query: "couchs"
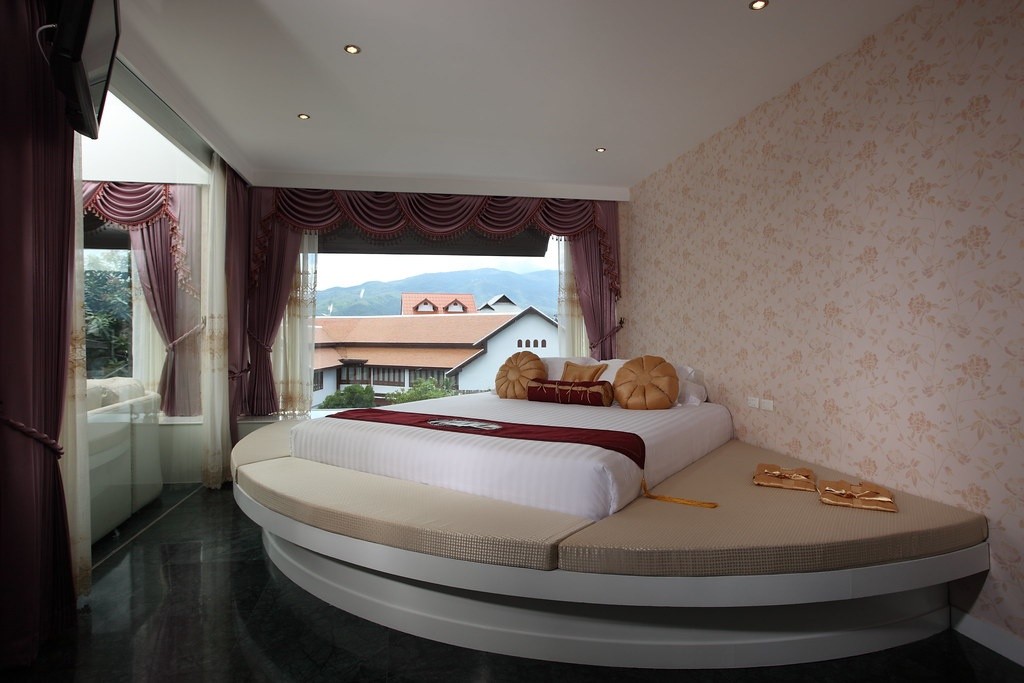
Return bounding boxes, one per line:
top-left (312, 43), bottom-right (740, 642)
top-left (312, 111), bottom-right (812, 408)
top-left (87, 376), bottom-right (161, 544)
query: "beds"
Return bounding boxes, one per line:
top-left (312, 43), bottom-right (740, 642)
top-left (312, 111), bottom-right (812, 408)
top-left (230, 354), bottom-right (991, 669)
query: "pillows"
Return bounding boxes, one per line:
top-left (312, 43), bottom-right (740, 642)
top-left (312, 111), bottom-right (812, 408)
top-left (613, 355), bottom-right (680, 409)
top-left (526, 378), bottom-right (614, 407)
top-left (495, 351), bottom-right (546, 399)
top-left (560, 361), bottom-right (608, 383)
